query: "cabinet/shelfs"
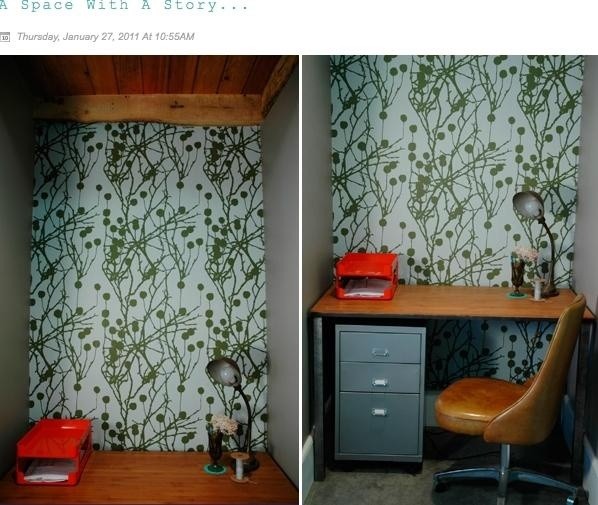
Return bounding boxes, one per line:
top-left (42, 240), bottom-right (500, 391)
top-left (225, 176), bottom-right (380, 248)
top-left (332, 325), bottom-right (426, 473)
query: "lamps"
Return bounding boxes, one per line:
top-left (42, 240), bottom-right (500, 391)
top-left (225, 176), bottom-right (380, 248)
top-left (511, 190), bottom-right (560, 299)
top-left (205, 358), bottom-right (261, 471)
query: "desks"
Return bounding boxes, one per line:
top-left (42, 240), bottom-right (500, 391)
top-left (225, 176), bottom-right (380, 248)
top-left (308, 275), bottom-right (595, 490)
top-left (1, 452), bottom-right (300, 505)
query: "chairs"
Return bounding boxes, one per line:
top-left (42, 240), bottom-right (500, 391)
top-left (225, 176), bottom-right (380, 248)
top-left (430, 288), bottom-right (593, 503)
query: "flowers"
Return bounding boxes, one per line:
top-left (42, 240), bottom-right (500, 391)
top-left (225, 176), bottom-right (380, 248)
top-left (512, 244), bottom-right (539, 266)
top-left (208, 414), bottom-right (238, 436)
